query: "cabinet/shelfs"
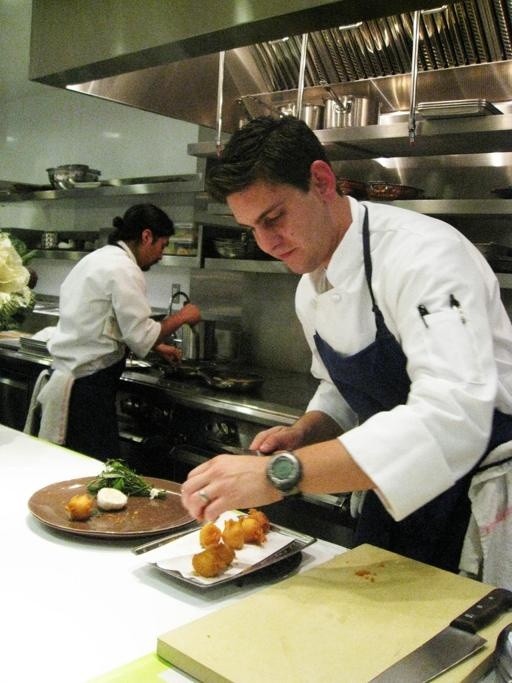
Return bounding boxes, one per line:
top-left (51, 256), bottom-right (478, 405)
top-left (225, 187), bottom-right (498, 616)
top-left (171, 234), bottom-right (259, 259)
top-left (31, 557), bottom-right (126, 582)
top-left (29, 0), bottom-right (511, 296)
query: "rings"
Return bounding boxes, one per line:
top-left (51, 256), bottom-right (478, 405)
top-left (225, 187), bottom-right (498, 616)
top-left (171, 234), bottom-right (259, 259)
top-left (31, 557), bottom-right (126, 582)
top-left (199, 491), bottom-right (211, 502)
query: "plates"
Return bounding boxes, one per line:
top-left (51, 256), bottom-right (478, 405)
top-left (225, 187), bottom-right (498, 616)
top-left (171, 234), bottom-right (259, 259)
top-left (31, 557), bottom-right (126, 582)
top-left (129, 512), bottom-right (318, 591)
top-left (73, 182), bottom-right (100, 190)
top-left (26, 475), bottom-right (207, 537)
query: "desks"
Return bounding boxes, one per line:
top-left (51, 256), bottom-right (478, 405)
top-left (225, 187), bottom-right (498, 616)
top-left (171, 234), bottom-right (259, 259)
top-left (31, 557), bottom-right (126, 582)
top-left (0, 421), bottom-right (512, 683)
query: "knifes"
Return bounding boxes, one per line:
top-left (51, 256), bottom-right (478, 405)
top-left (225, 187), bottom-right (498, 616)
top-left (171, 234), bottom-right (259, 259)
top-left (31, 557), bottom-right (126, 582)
top-left (367, 588), bottom-right (512, 683)
top-left (220, 446), bottom-right (273, 458)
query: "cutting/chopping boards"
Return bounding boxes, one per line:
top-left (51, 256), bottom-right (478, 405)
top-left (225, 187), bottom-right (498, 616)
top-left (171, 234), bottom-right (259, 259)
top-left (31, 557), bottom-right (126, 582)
top-left (157, 543), bottom-right (512, 683)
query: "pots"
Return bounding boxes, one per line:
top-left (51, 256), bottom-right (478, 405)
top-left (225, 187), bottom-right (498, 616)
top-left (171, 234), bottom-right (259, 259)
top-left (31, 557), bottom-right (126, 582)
top-left (369, 181), bottom-right (424, 201)
top-left (151, 357), bottom-right (265, 392)
top-left (243, 80), bottom-right (378, 130)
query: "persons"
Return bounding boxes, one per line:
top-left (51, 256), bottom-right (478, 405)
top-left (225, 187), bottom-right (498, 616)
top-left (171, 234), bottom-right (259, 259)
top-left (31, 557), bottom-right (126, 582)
top-left (178, 110), bottom-right (510, 596)
top-left (20, 202), bottom-right (204, 462)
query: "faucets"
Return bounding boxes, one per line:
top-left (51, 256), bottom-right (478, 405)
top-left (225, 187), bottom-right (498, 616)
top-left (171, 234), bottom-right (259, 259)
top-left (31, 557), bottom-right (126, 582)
top-left (169, 292), bottom-right (190, 316)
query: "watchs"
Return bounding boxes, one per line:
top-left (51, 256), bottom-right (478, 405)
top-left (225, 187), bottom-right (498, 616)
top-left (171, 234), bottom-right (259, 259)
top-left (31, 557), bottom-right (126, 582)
top-left (265, 450), bottom-right (304, 504)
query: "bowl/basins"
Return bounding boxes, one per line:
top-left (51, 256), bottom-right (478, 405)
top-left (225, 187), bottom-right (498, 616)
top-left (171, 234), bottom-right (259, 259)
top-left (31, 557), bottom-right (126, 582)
top-left (212, 236), bottom-right (272, 261)
top-left (44, 166), bottom-right (101, 190)
